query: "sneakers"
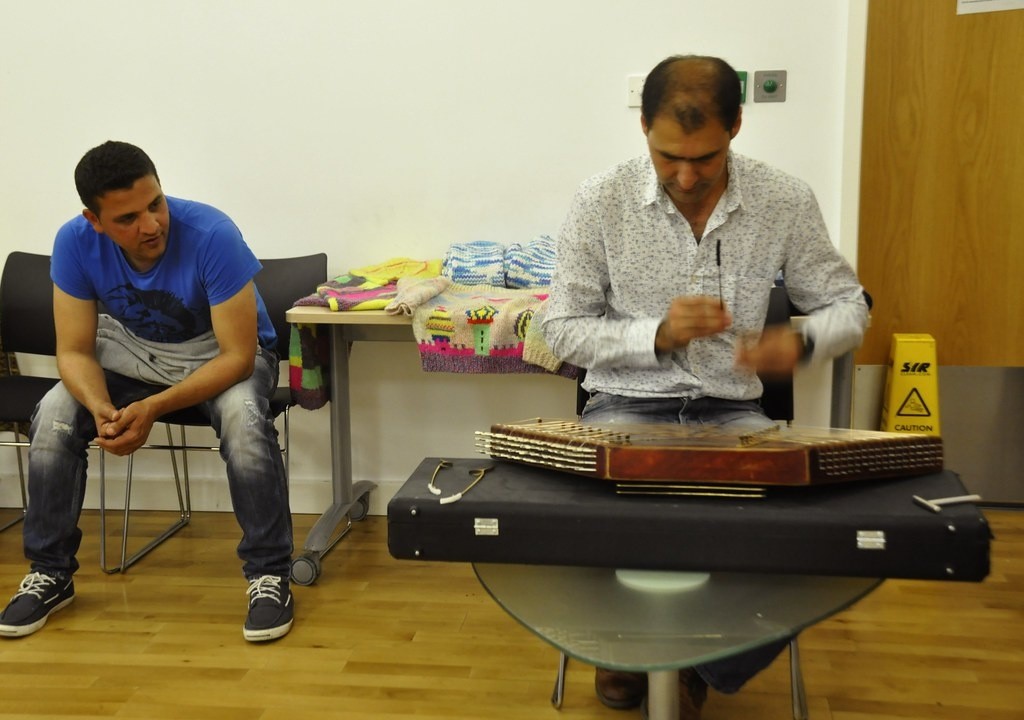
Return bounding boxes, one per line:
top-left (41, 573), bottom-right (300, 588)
top-left (0, 568), bottom-right (76, 637)
top-left (243, 576), bottom-right (295, 642)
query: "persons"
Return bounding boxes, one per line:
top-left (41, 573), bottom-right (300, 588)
top-left (1, 140), bottom-right (294, 641)
top-left (545, 54), bottom-right (871, 720)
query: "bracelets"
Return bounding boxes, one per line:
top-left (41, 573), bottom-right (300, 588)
top-left (800, 336), bottom-right (813, 363)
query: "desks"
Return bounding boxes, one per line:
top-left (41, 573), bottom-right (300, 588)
top-left (285, 306), bottom-right (856, 587)
top-left (473, 562), bottom-right (884, 720)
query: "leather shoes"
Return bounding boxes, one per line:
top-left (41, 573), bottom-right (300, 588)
top-left (595, 666), bottom-right (707, 720)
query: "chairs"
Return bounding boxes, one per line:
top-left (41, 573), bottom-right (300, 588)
top-left (553, 287), bottom-right (809, 720)
top-left (0, 252), bottom-right (185, 575)
top-left (119, 253), bottom-right (328, 574)
